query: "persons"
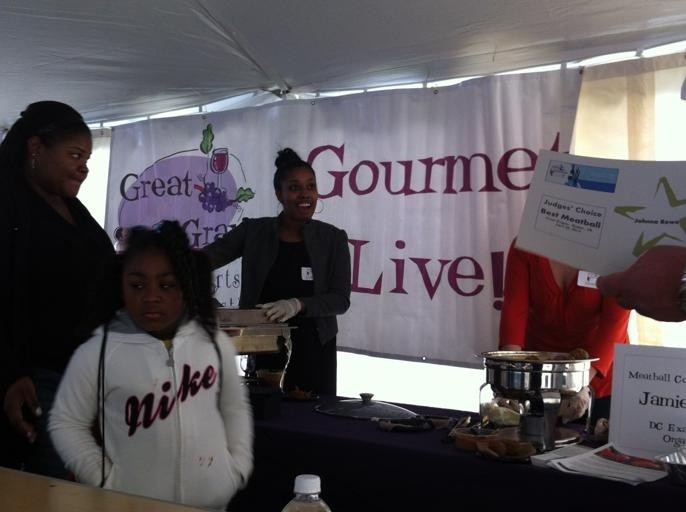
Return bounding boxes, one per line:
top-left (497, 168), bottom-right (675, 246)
top-left (597, 244), bottom-right (686, 323)
top-left (45, 218), bottom-right (255, 511)
top-left (0, 100), bottom-right (122, 483)
top-left (492, 232), bottom-right (632, 434)
top-left (201, 146), bottom-right (353, 396)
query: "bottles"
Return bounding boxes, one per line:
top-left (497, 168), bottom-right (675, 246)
top-left (282, 471), bottom-right (333, 512)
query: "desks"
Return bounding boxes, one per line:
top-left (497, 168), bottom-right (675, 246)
top-left (243, 376), bottom-right (686, 510)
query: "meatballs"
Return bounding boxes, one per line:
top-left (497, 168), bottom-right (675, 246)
top-left (569, 348), bottom-right (591, 360)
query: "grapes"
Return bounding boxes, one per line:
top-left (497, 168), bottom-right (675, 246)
top-left (199, 182), bottom-right (232, 213)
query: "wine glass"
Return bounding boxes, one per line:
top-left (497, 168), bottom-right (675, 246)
top-left (210, 147), bottom-right (229, 193)
